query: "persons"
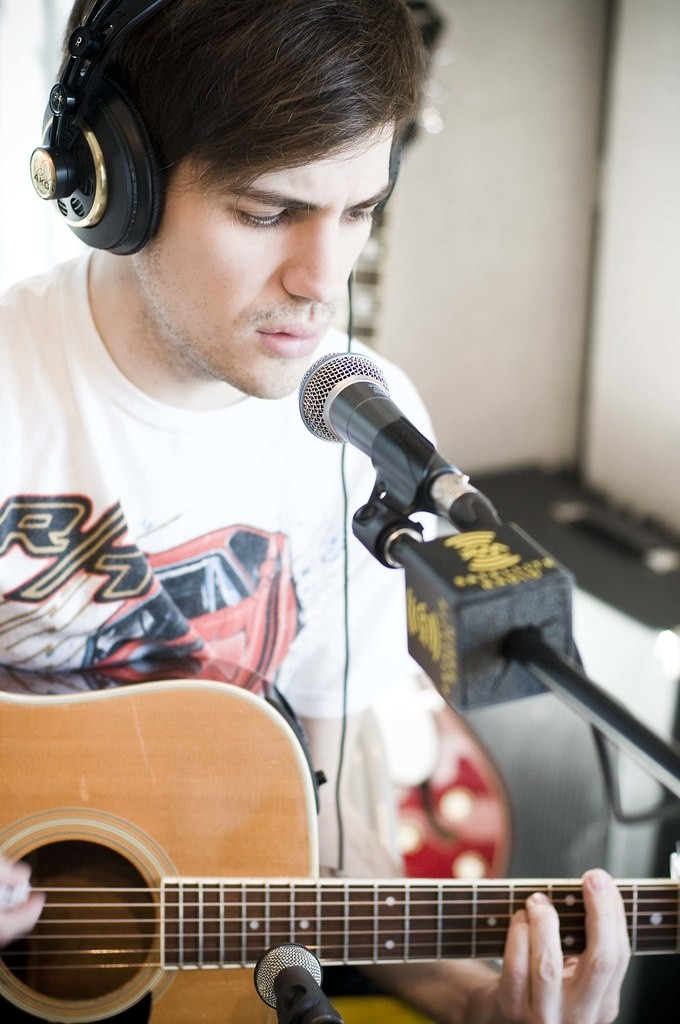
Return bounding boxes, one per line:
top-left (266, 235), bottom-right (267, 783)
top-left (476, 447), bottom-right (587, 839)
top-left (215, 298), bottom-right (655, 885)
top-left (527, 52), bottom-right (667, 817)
top-left (0, 1), bottom-right (636, 1024)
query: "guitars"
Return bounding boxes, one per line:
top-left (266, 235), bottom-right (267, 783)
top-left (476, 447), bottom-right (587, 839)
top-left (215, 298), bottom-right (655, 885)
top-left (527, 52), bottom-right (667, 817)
top-left (0, 647), bottom-right (680, 1024)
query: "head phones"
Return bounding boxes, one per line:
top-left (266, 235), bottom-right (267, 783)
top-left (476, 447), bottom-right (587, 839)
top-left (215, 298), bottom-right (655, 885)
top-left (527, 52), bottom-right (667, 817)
top-left (30, 0), bottom-right (172, 257)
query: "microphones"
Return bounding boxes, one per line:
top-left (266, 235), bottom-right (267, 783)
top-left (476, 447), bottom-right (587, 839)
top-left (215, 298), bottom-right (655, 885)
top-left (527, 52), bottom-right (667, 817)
top-left (253, 942), bottom-right (343, 1024)
top-left (296, 352), bottom-right (501, 530)
